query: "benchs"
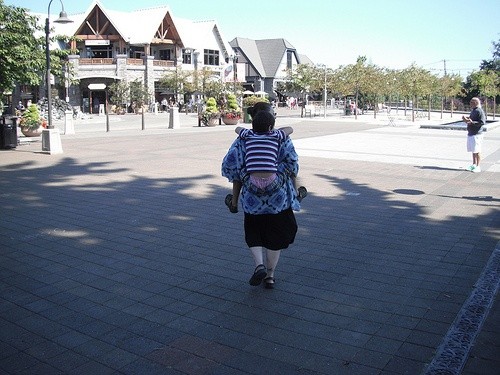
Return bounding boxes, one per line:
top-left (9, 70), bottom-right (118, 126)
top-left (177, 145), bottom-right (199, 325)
top-left (305, 104), bottom-right (321, 119)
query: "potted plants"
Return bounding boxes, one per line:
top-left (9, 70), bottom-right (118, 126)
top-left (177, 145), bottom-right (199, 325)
top-left (221, 94), bottom-right (241, 125)
top-left (200, 97), bottom-right (221, 127)
top-left (18, 104), bottom-right (47, 137)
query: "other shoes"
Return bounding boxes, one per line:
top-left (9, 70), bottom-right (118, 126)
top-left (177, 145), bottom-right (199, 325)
top-left (296, 186), bottom-right (307, 203)
top-left (472, 166), bottom-right (480, 172)
top-left (249, 264), bottom-right (267, 286)
top-left (264, 277), bottom-right (277, 289)
top-left (465, 164), bottom-right (476, 171)
top-left (225, 193), bottom-right (238, 213)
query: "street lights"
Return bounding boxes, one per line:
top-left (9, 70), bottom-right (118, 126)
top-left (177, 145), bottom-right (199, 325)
top-left (322, 64), bottom-right (327, 117)
top-left (42, 0), bottom-right (74, 155)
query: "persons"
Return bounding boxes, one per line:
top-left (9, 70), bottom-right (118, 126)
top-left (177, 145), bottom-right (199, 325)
top-left (16, 100), bottom-right (32, 110)
top-left (220, 102), bottom-right (302, 289)
top-left (151, 96), bottom-right (357, 113)
top-left (462, 97), bottom-right (486, 174)
top-left (225, 110), bottom-right (308, 214)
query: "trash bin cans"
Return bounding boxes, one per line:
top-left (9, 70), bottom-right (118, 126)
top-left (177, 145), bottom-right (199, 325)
top-left (243, 105), bottom-right (253, 123)
top-left (0, 116), bottom-right (18, 149)
